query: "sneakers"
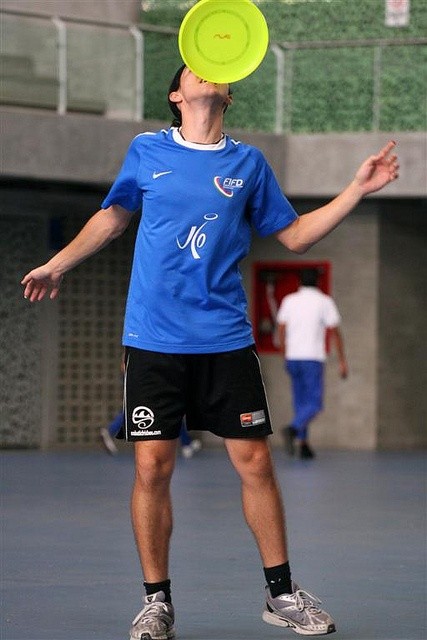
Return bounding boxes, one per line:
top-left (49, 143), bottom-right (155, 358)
top-left (262, 581), bottom-right (336, 636)
top-left (129, 590), bottom-right (176, 639)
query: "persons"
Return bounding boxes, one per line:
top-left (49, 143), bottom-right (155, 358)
top-left (99, 349), bottom-right (202, 459)
top-left (274, 267), bottom-right (348, 459)
top-left (20, 64), bottom-right (400, 640)
